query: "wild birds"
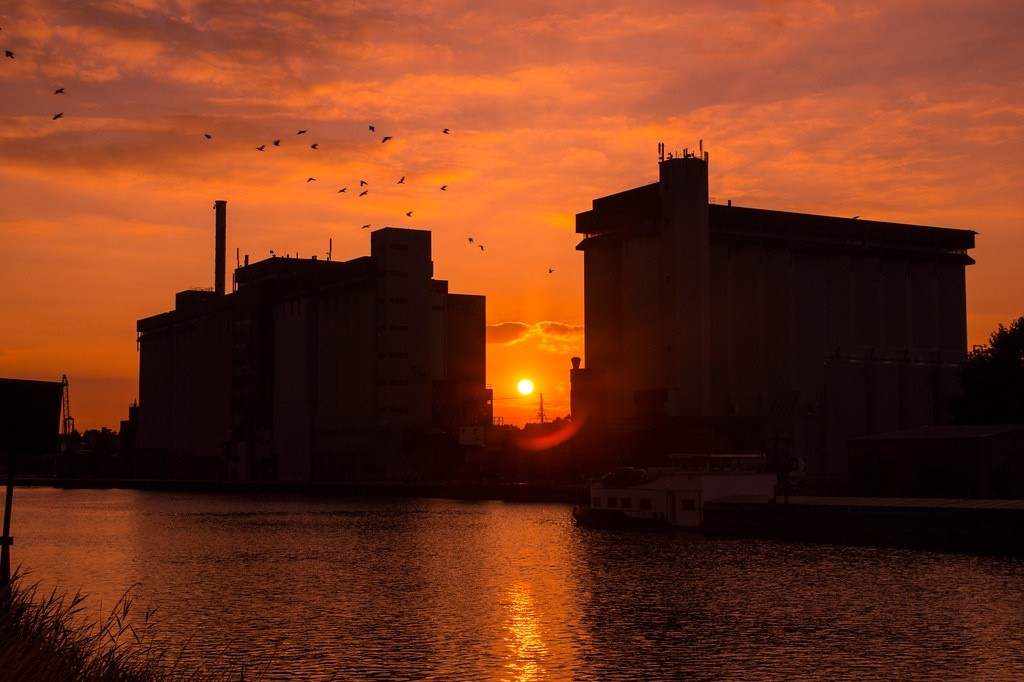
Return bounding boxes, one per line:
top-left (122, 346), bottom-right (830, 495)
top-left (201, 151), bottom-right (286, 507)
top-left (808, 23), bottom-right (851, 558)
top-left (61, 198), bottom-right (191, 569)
top-left (467, 237), bottom-right (555, 275)
top-left (296, 130), bottom-right (308, 135)
top-left (442, 128), bottom-right (450, 134)
top-left (338, 187), bottom-right (347, 193)
top-left (255, 145), bottom-right (265, 152)
top-left (440, 185), bottom-right (448, 191)
top-left (307, 177), bottom-right (316, 183)
top-left (53, 112), bottom-right (65, 120)
top-left (406, 211), bottom-right (412, 217)
top-left (362, 224), bottom-right (371, 228)
top-left (204, 133), bottom-right (212, 139)
top-left (397, 176), bottom-right (406, 184)
top-left (273, 139), bottom-right (280, 146)
top-left (359, 190), bottom-right (369, 197)
top-left (360, 180), bottom-right (368, 186)
top-left (4, 50), bottom-right (15, 59)
top-left (382, 137), bottom-right (393, 143)
top-left (311, 144), bottom-right (318, 149)
top-left (53, 87), bottom-right (66, 94)
top-left (369, 125), bottom-right (376, 133)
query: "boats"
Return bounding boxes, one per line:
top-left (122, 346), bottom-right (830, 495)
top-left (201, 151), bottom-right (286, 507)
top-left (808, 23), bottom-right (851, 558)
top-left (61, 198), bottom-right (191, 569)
top-left (572, 453), bottom-right (777, 531)
